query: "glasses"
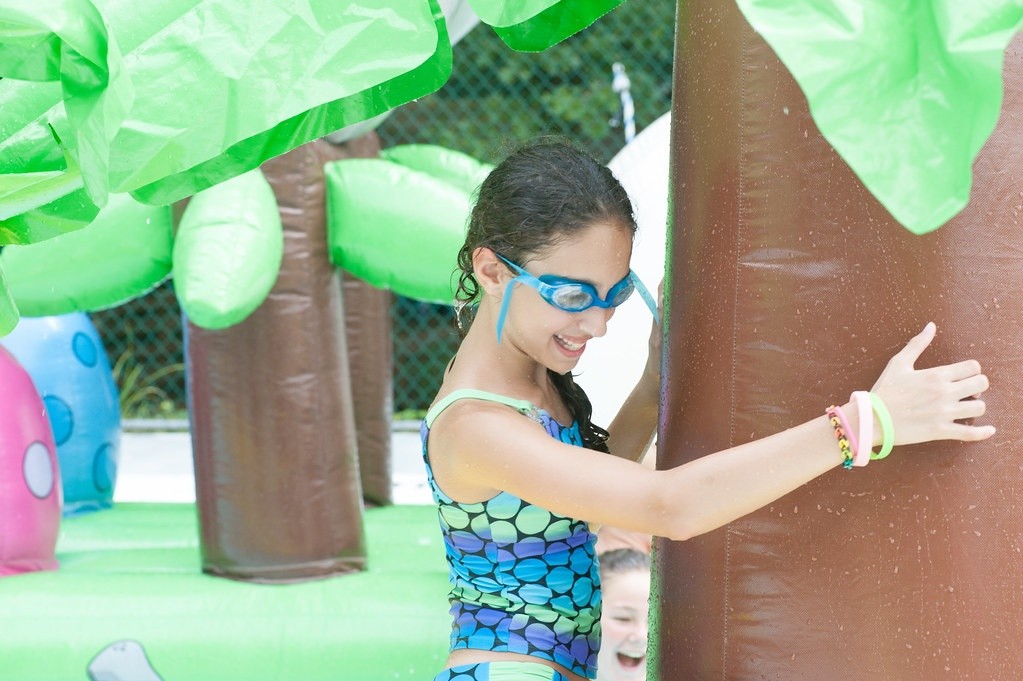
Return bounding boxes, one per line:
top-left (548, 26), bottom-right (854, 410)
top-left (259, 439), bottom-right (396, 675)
top-left (495, 252), bottom-right (636, 313)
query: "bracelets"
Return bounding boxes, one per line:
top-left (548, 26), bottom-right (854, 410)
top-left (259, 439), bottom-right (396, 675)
top-left (827, 390), bottom-right (895, 470)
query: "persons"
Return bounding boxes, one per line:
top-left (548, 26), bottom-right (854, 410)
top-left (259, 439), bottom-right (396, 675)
top-left (418, 142), bottom-right (996, 680)
top-left (590, 547), bottom-right (653, 681)
